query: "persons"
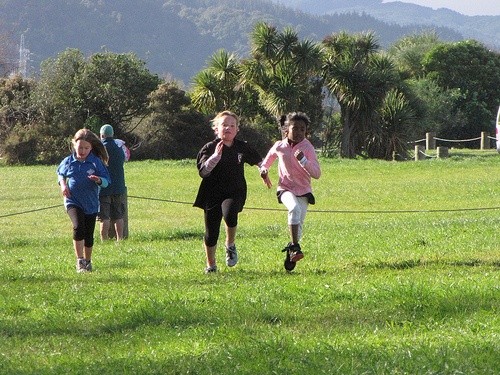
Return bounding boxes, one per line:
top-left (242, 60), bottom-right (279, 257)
top-left (260, 111), bottom-right (322, 271)
top-left (93, 123), bottom-right (131, 242)
top-left (191, 111), bottom-right (269, 271)
top-left (57, 129), bottom-right (111, 273)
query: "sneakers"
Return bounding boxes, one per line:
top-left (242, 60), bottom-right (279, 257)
top-left (284, 241), bottom-right (304, 271)
top-left (206, 266), bottom-right (217, 273)
top-left (76, 258), bottom-right (93, 274)
top-left (225, 245), bottom-right (238, 267)
